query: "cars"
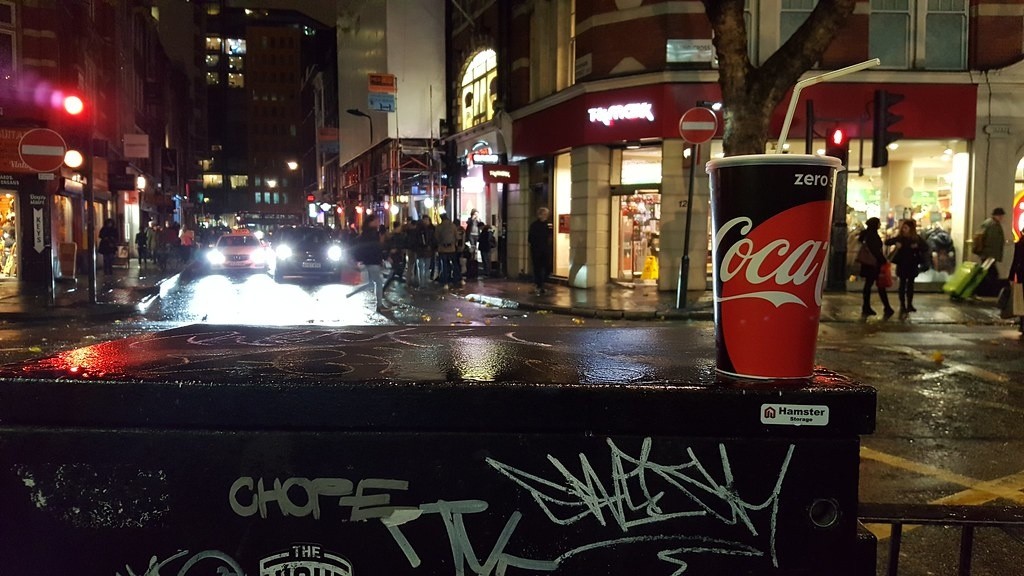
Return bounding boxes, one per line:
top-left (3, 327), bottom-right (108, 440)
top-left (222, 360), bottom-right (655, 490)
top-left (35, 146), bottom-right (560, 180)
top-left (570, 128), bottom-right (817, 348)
top-left (209, 229), bottom-right (265, 271)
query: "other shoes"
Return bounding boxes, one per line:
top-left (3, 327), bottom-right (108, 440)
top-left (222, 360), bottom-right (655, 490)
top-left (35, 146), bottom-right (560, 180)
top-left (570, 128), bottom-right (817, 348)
top-left (535, 285), bottom-right (547, 295)
top-left (907, 306), bottom-right (917, 312)
top-left (861, 307), bottom-right (877, 315)
top-left (900, 307), bottom-right (908, 313)
top-left (883, 308), bottom-right (894, 314)
top-left (377, 305), bottom-right (391, 313)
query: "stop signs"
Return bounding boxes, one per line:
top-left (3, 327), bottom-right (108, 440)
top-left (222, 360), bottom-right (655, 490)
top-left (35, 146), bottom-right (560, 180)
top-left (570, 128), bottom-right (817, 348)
top-left (18, 127), bottom-right (68, 172)
top-left (680, 107), bottom-right (718, 145)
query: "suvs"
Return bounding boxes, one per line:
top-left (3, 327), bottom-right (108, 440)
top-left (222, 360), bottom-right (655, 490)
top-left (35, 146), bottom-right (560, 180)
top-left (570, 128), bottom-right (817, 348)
top-left (271, 224), bottom-right (342, 282)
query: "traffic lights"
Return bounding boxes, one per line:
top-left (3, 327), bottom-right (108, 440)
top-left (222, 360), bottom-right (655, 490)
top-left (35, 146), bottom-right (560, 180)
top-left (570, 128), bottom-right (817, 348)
top-left (61, 90), bottom-right (89, 127)
top-left (872, 89), bottom-right (904, 167)
top-left (826, 125), bottom-right (850, 164)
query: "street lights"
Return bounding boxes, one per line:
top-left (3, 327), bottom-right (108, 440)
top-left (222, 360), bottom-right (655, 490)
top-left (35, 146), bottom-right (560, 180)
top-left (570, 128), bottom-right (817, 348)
top-left (347, 106), bottom-right (374, 146)
top-left (285, 158), bottom-right (306, 224)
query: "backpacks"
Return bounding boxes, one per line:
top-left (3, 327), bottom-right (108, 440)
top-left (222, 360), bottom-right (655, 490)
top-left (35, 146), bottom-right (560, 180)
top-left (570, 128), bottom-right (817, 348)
top-left (972, 219), bottom-right (995, 251)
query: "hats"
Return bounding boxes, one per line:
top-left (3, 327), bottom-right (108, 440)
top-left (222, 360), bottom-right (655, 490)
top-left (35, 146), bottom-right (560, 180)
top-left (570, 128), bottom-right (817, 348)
top-left (992, 207), bottom-right (1007, 217)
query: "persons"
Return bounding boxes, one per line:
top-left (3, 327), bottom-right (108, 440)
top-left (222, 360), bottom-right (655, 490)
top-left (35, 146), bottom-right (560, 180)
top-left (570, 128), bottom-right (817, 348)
top-left (345, 214), bottom-right (395, 312)
top-left (885, 220), bottom-right (929, 313)
top-left (98, 219), bottom-right (120, 274)
top-left (1008, 228), bottom-right (1024, 284)
top-left (379, 207), bottom-right (497, 291)
top-left (135, 216), bottom-right (198, 273)
top-left (527, 207), bottom-right (562, 296)
top-left (858, 217), bottom-right (894, 315)
top-left (970, 207), bottom-right (1007, 300)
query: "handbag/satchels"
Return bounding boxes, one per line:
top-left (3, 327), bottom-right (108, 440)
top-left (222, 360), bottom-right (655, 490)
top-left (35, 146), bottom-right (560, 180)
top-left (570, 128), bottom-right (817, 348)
top-left (850, 253), bottom-right (863, 276)
top-left (876, 263), bottom-right (892, 289)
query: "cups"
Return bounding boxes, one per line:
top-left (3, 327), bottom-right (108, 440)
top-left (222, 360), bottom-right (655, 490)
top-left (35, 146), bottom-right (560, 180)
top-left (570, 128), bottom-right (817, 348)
top-left (705, 154), bottom-right (846, 381)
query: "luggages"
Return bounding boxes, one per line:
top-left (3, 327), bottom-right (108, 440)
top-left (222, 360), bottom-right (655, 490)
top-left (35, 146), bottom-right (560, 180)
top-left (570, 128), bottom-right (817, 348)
top-left (942, 254), bottom-right (996, 301)
